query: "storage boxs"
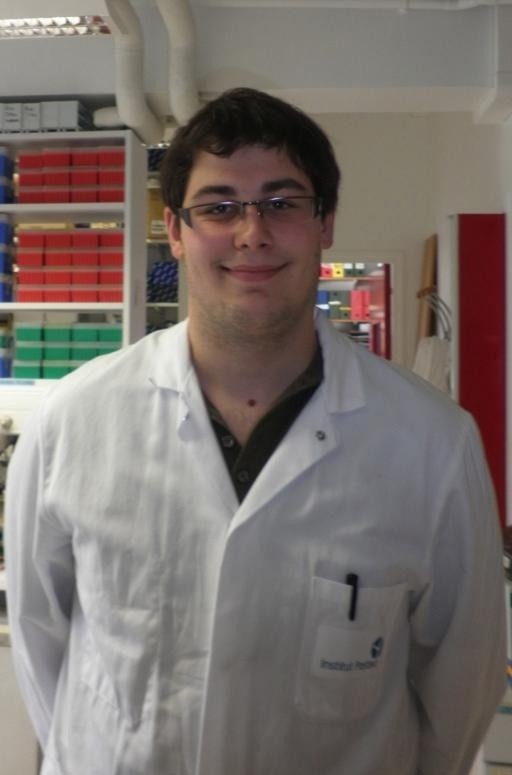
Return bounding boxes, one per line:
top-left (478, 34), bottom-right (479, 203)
top-left (316, 262), bottom-right (369, 323)
top-left (2, 99), bottom-right (92, 129)
top-left (1, 143), bottom-right (124, 383)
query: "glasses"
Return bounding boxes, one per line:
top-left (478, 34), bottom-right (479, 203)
top-left (178, 196), bottom-right (318, 235)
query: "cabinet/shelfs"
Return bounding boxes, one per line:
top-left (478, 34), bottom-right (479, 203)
top-left (140, 145), bottom-right (188, 334)
top-left (0, 129), bottom-right (147, 648)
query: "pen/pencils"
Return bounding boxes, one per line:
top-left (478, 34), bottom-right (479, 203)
top-left (346, 572), bottom-right (359, 624)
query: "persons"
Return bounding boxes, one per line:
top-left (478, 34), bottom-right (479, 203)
top-left (3, 87), bottom-right (501, 775)
